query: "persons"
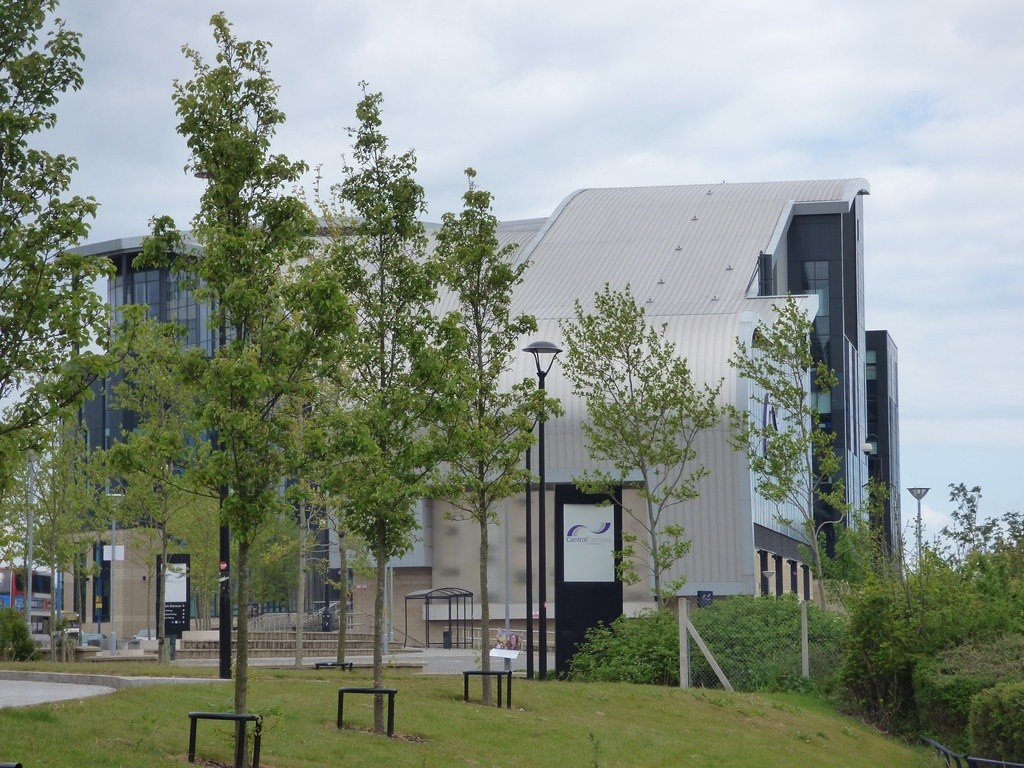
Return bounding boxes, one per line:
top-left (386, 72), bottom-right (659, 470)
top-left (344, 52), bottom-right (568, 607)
top-left (496, 634), bottom-right (518, 649)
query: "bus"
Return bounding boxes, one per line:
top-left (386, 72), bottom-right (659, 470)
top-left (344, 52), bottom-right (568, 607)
top-left (0, 566), bottom-right (54, 632)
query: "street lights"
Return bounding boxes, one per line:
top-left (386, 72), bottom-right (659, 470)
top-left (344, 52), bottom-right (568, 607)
top-left (903, 485), bottom-right (931, 569)
top-left (105, 492), bottom-right (127, 656)
top-left (762, 571), bottom-right (775, 596)
top-left (521, 340), bottom-right (564, 682)
